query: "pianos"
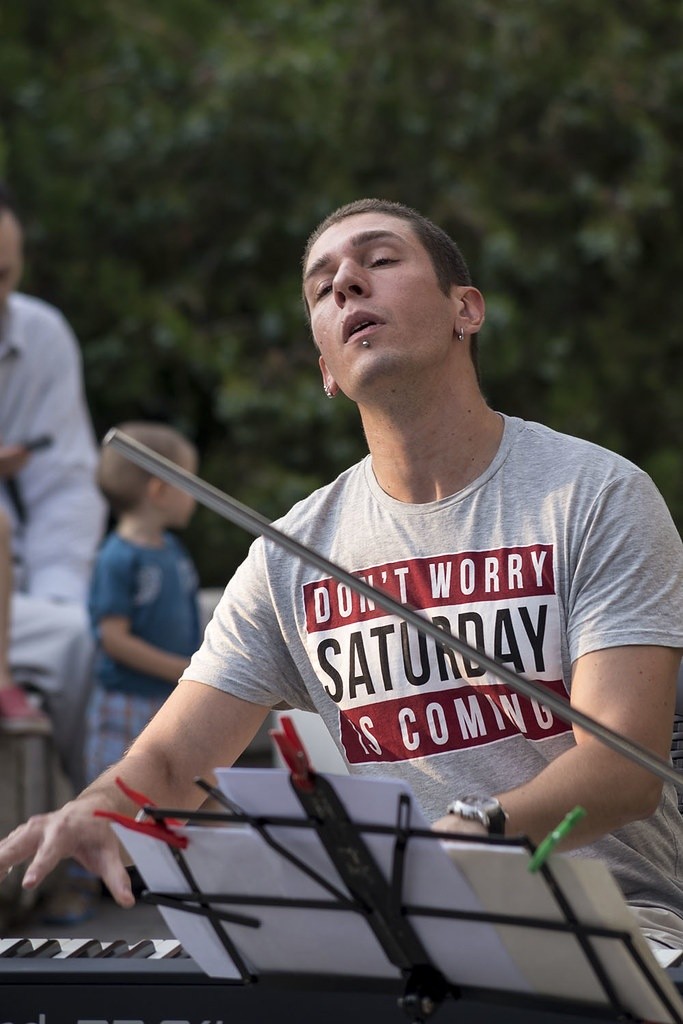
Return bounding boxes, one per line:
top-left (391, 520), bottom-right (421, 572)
top-left (0, 933), bottom-right (683, 1024)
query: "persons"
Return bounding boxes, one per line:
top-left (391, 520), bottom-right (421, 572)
top-left (0, 207), bottom-right (108, 782)
top-left (0, 197), bottom-right (683, 982)
top-left (84, 424), bottom-right (201, 794)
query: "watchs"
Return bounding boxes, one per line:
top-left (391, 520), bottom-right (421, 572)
top-left (447, 794), bottom-right (505, 840)
